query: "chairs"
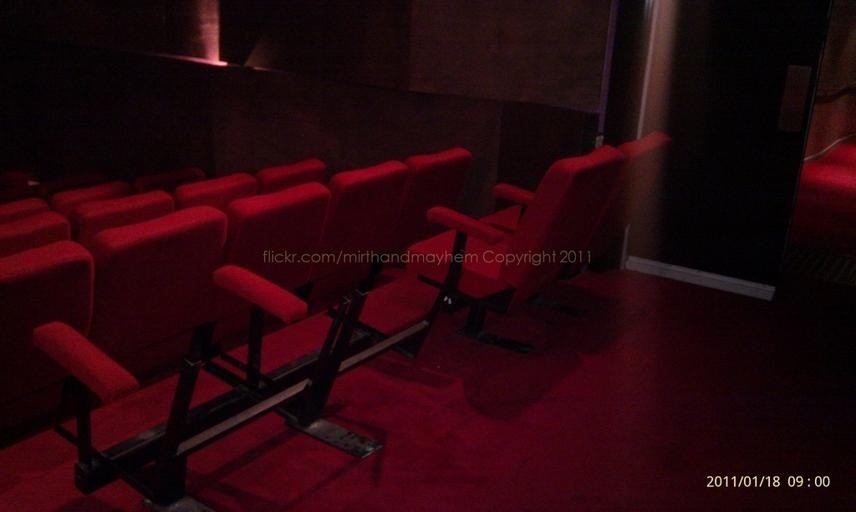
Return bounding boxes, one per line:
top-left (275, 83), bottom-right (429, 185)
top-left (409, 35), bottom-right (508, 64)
top-left (492, 130), bottom-right (680, 324)
top-left (1, 124), bottom-right (484, 509)
top-left (405, 146), bottom-right (622, 367)
